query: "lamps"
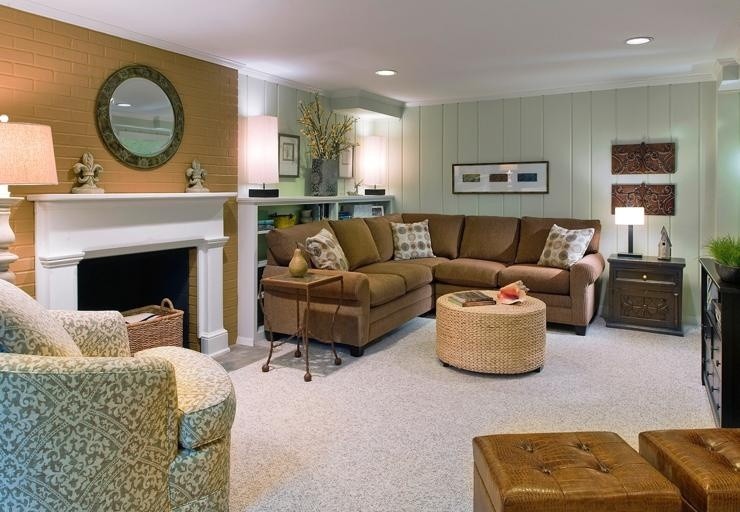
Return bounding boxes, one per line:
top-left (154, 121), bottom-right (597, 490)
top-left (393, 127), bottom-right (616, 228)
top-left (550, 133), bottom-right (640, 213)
top-left (246, 114), bottom-right (280, 197)
top-left (0, 114), bottom-right (59, 287)
top-left (615, 207), bottom-right (644, 257)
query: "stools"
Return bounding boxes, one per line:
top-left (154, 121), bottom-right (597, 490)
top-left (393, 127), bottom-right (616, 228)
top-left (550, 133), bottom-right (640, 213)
top-left (472, 431), bottom-right (682, 512)
top-left (639, 428), bottom-right (740, 512)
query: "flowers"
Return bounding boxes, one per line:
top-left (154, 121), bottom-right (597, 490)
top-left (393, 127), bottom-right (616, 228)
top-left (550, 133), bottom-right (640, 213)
top-left (295, 86), bottom-right (361, 159)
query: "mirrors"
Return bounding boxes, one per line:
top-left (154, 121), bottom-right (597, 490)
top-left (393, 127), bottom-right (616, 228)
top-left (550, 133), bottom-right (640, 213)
top-left (96, 65), bottom-right (185, 168)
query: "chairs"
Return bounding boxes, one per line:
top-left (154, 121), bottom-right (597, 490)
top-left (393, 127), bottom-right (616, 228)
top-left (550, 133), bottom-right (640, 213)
top-left (0, 278), bottom-right (235, 511)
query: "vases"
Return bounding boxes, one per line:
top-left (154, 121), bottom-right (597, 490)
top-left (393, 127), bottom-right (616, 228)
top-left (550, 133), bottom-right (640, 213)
top-left (311, 159), bottom-right (339, 196)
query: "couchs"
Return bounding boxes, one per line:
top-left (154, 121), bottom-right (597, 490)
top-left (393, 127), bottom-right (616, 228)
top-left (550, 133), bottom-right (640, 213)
top-left (259, 212), bottom-right (606, 355)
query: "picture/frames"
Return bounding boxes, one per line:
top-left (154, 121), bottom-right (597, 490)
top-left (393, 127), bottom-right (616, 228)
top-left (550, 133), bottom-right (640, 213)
top-left (452, 160), bottom-right (548, 194)
top-left (338, 143), bottom-right (353, 179)
top-left (278, 133), bottom-right (299, 177)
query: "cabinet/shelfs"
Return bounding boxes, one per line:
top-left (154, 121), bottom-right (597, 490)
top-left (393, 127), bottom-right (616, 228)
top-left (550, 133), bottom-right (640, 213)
top-left (697, 258), bottom-right (740, 428)
top-left (606, 254), bottom-right (686, 337)
top-left (237, 194), bottom-right (394, 347)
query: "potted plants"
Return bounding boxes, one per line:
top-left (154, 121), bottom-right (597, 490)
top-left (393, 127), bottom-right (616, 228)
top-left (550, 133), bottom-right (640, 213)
top-left (703, 232), bottom-right (739, 282)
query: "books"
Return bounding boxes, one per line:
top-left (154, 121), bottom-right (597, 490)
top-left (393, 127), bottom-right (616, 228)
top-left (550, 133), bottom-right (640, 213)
top-left (447, 297), bottom-right (496, 306)
top-left (451, 290), bottom-right (493, 303)
